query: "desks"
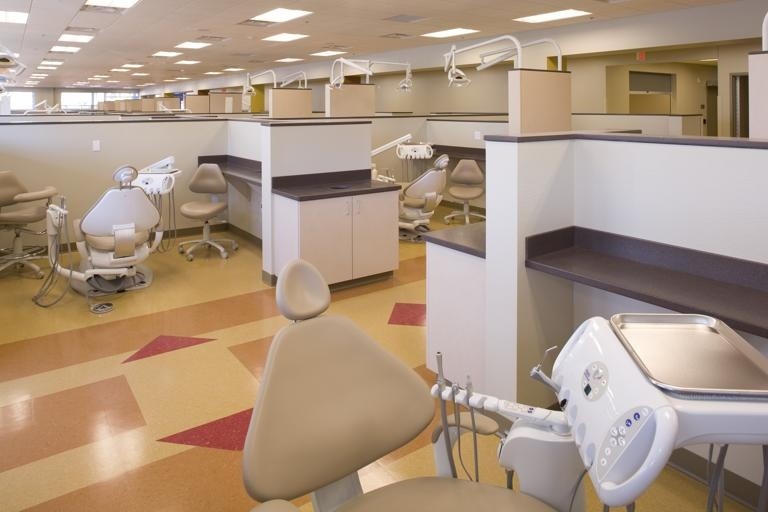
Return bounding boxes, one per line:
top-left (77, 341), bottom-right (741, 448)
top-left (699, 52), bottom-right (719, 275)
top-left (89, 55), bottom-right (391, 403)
top-left (198, 155), bottom-right (261, 184)
top-left (526, 244), bottom-right (768, 338)
top-left (429, 145), bottom-right (485, 160)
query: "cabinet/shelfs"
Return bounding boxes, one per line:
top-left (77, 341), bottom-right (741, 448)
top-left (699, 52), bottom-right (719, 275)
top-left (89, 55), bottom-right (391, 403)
top-left (270, 188), bottom-right (398, 291)
top-left (425, 245), bottom-right (487, 394)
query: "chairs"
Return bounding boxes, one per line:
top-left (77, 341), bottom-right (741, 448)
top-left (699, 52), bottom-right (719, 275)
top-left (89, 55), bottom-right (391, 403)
top-left (241, 258), bottom-right (561, 511)
top-left (444, 158), bottom-right (486, 224)
top-left (0, 154), bottom-right (240, 297)
top-left (392, 153), bottom-right (450, 242)
